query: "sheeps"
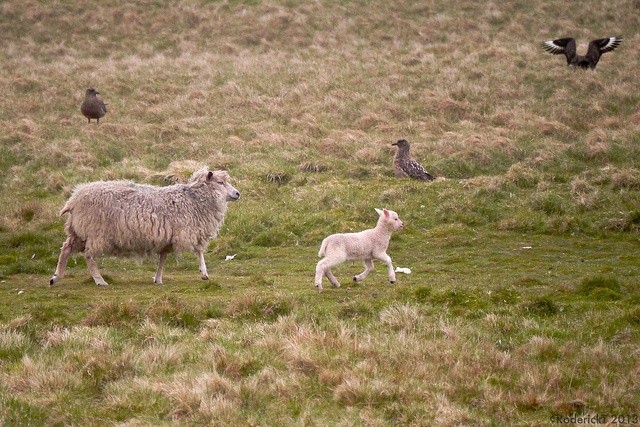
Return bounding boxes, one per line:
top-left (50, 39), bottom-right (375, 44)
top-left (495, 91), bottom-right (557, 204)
top-left (312, 205), bottom-right (406, 292)
top-left (48, 169), bottom-right (240, 289)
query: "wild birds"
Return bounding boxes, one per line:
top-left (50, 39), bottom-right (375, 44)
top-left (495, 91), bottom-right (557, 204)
top-left (390, 138), bottom-right (433, 183)
top-left (80, 87), bottom-right (106, 124)
top-left (534, 32), bottom-right (624, 69)
